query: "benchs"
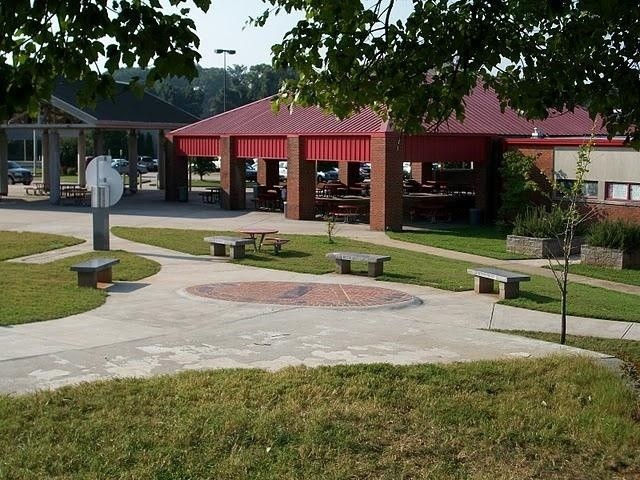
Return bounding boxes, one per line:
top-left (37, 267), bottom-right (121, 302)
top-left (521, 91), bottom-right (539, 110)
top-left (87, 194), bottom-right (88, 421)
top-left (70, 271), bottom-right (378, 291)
top-left (325, 252), bottom-right (391, 278)
top-left (70, 258), bottom-right (121, 289)
top-left (466, 266), bottom-right (531, 300)
top-left (205, 235), bottom-right (254, 260)
top-left (199, 186), bottom-right (221, 204)
top-left (233, 229), bottom-right (289, 255)
top-left (315, 178), bottom-right (449, 223)
top-left (24, 181), bottom-right (91, 206)
top-left (250, 184), bottom-right (286, 213)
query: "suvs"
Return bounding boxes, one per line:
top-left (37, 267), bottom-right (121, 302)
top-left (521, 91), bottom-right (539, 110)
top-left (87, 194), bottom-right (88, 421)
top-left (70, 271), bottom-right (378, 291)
top-left (7, 160), bottom-right (34, 185)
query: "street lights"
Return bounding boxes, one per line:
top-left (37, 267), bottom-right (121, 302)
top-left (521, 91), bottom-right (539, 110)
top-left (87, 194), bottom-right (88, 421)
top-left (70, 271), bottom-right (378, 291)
top-left (214, 49), bottom-right (237, 113)
